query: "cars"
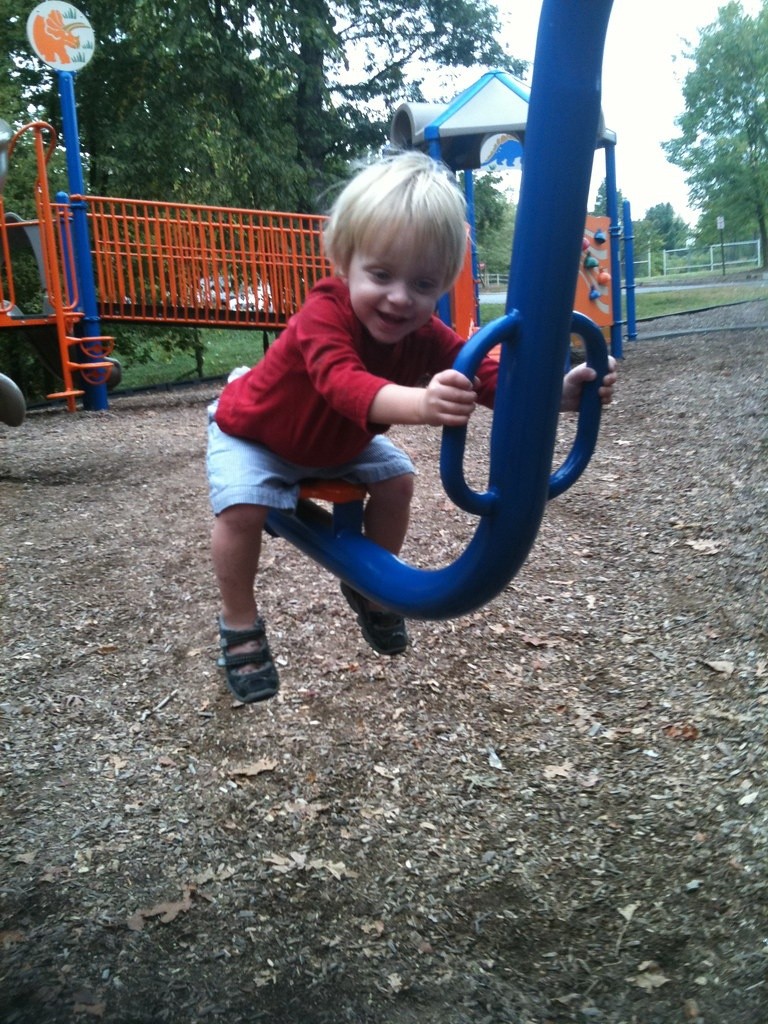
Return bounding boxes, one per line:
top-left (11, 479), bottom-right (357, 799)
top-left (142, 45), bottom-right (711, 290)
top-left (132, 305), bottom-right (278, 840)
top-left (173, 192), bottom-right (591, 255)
top-left (197, 274), bottom-right (273, 312)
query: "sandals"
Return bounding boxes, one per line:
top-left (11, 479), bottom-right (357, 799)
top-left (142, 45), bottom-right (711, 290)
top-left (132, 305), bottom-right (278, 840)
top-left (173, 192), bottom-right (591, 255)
top-left (340, 581), bottom-right (408, 654)
top-left (217, 614), bottom-right (279, 703)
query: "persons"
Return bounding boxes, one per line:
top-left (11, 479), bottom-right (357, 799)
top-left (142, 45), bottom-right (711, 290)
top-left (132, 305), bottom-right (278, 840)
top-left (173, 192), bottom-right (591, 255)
top-left (206, 149), bottom-right (619, 704)
